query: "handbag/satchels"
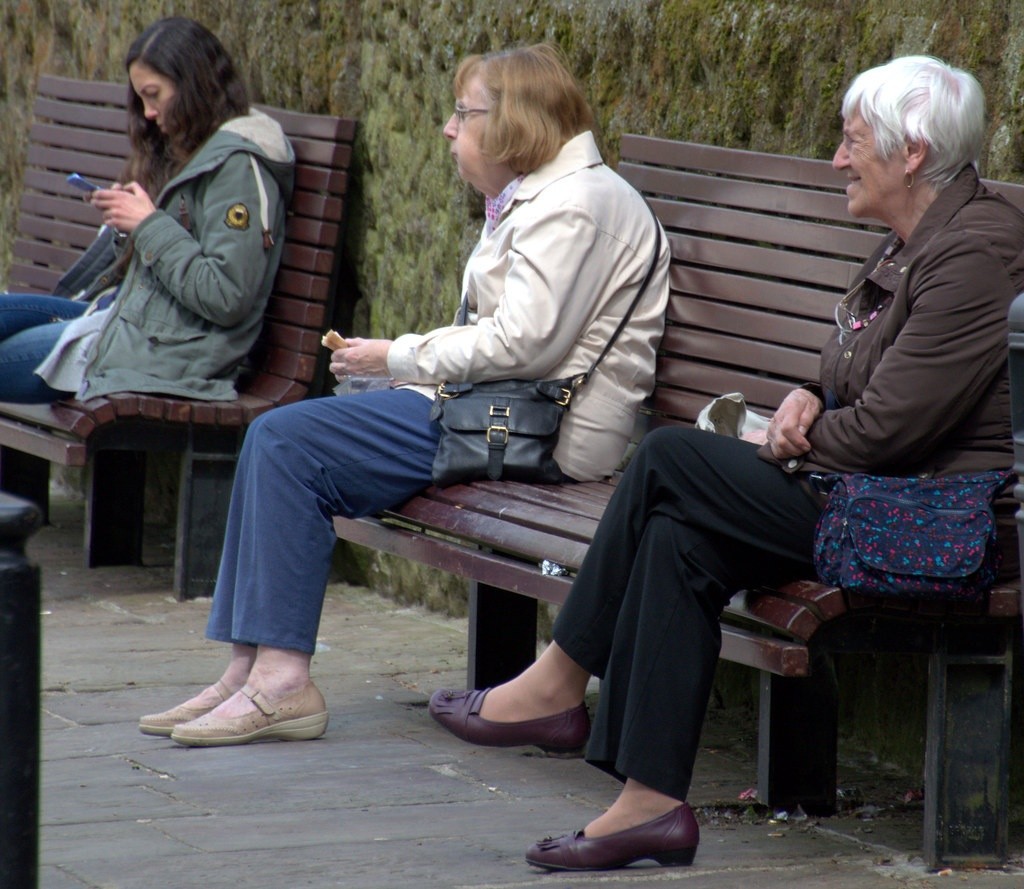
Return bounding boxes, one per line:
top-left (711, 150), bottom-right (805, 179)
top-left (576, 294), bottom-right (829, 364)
top-left (430, 374), bottom-right (573, 490)
top-left (814, 471), bottom-right (1013, 617)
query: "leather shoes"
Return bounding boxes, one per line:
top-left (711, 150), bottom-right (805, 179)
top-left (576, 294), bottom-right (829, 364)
top-left (428, 687), bottom-right (591, 753)
top-left (524, 803), bottom-right (699, 871)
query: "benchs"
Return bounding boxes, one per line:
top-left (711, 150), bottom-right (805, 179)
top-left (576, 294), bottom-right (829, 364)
top-left (0, 76), bottom-right (358, 572)
top-left (333, 131), bottom-right (1024, 814)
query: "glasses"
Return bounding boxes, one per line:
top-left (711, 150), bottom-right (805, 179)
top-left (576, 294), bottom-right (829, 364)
top-left (454, 102), bottom-right (489, 123)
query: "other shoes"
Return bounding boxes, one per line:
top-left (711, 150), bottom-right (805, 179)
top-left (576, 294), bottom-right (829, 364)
top-left (139, 679), bottom-right (232, 736)
top-left (170, 682), bottom-right (329, 747)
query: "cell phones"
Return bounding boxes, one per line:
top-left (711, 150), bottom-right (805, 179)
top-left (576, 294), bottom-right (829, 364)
top-left (66, 173), bottom-right (102, 192)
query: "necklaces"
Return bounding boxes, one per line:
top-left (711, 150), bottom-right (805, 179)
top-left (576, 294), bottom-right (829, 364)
top-left (833, 237), bottom-right (900, 342)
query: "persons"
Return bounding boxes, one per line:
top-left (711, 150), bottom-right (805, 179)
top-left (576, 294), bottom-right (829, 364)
top-left (138, 42), bottom-right (671, 749)
top-left (0, 18), bottom-right (297, 404)
top-left (428, 53), bottom-right (1023, 872)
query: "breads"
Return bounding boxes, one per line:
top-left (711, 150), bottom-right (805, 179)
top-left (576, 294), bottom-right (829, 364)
top-left (321, 329), bottom-right (348, 351)
